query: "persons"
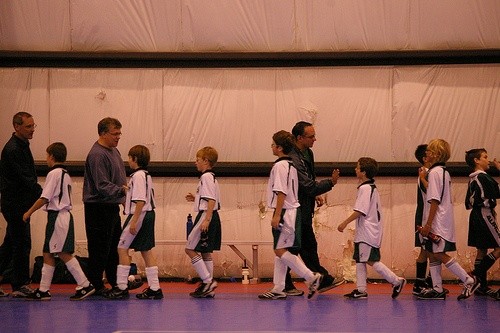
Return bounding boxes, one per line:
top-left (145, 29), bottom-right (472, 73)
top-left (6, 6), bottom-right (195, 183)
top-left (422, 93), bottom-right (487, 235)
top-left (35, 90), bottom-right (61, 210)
top-left (257, 130), bottom-right (323, 299)
top-left (279, 120), bottom-right (345, 296)
top-left (464, 148), bottom-right (500, 298)
top-left (23, 142), bottom-right (95, 302)
top-left (107, 144), bottom-right (164, 299)
top-left (415, 139), bottom-right (480, 300)
top-left (336, 157), bottom-right (406, 299)
top-left (82, 116), bottom-right (127, 298)
top-left (0, 112), bottom-right (44, 298)
top-left (412, 144), bottom-right (449, 296)
top-left (185, 146), bottom-right (222, 298)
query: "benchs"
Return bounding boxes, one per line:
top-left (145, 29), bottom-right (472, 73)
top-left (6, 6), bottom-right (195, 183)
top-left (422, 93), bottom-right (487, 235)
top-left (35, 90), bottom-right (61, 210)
top-left (155, 240), bottom-right (274, 284)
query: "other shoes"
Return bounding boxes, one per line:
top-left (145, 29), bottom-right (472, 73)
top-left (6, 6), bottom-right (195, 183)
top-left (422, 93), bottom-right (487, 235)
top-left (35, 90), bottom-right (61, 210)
top-left (95, 287), bottom-right (109, 295)
top-left (128, 281), bottom-right (142, 289)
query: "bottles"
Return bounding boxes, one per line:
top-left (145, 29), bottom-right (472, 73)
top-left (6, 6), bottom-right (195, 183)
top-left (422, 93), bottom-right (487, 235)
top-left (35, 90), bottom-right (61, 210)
top-left (417, 226), bottom-right (441, 244)
top-left (241, 267), bottom-right (250, 285)
top-left (186, 213), bottom-right (193, 240)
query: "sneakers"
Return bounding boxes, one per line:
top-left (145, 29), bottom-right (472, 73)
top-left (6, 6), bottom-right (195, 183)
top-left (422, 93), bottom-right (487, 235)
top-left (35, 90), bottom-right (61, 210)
top-left (11, 285), bottom-right (34, 297)
top-left (100, 286), bottom-right (129, 299)
top-left (0, 289), bottom-right (9, 297)
top-left (318, 277), bottom-right (344, 293)
top-left (287, 288), bottom-right (304, 296)
top-left (308, 272), bottom-right (323, 298)
top-left (344, 289), bottom-right (368, 299)
top-left (24, 288), bottom-right (51, 300)
top-left (196, 280), bottom-right (217, 298)
top-left (69, 283), bottom-right (96, 301)
top-left (416, 288), bottom-right (445, 300)
top-left (468, 272), bottom-right (485, 295)
top-left (258, 289), bottom-right (287, 300)
top-left (473, 285), bottom-right (497, 298)
top-left (136, 287), bottom-right (163, 299)
top-left (190, 290), bottom-right (215, 298)
top-left (458, 277), bottom-right (481, 301)
top-left (392, 277), bottom-right (406, 299)
top-left (412, 277), bottom-right (449, 295)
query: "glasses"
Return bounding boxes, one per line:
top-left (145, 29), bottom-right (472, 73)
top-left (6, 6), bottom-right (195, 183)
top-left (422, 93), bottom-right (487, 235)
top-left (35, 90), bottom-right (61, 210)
top-left (22, 123), bottom-right (37, 130)
top-left (107, 132), bottom-right (122, 137)
